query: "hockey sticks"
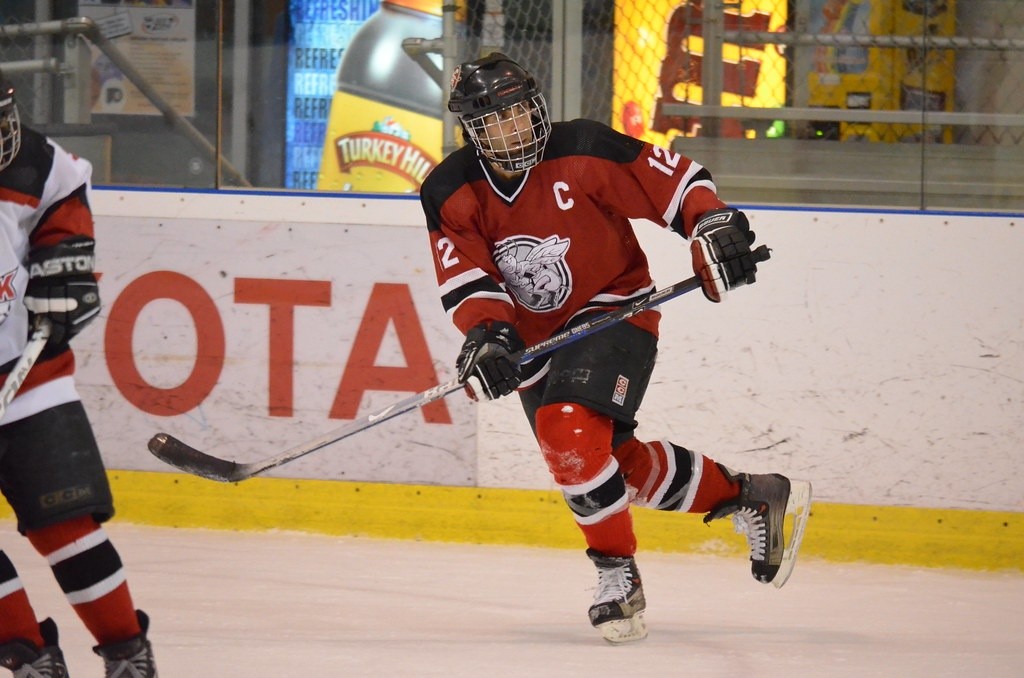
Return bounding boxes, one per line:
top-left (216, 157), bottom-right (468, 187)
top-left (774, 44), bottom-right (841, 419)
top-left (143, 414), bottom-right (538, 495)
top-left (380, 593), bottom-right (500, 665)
top-left (146, 245), bottom-right (772, 483)
top-left (0, 316), bottom-right (53, 420)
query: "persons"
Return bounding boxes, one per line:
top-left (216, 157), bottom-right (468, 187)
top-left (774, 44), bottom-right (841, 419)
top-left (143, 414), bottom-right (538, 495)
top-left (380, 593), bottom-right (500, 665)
top-left (0, 71), bottom-right (156, 678)
top-left (420, 52), bottom-right (813, 644)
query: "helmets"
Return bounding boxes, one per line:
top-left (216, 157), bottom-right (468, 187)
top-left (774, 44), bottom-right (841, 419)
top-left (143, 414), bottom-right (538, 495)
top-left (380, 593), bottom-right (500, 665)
top-left (0, 71), bottom-right (20, 172)
top-left (447, 52), bottom-right (552, 171)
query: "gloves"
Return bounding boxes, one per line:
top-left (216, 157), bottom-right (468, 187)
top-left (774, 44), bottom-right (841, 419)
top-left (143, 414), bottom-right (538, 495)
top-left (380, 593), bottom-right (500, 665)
top-left (456, 321), bottom-right (526, 401)
top-left (691, 209), bottom-right (758, 303)
top-left (22, 238), bottom-right (102, 340)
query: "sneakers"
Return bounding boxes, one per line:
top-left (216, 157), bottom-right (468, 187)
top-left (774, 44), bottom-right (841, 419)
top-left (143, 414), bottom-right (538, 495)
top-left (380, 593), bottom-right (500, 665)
top-left (703, 462), bottom-right (791, 584)
top-left (586, 548), bottom-right (646, 626)
top-left (0, 617), bottom-right (69, 678)
top-left (92, 609), bottom-right (157, 678)
